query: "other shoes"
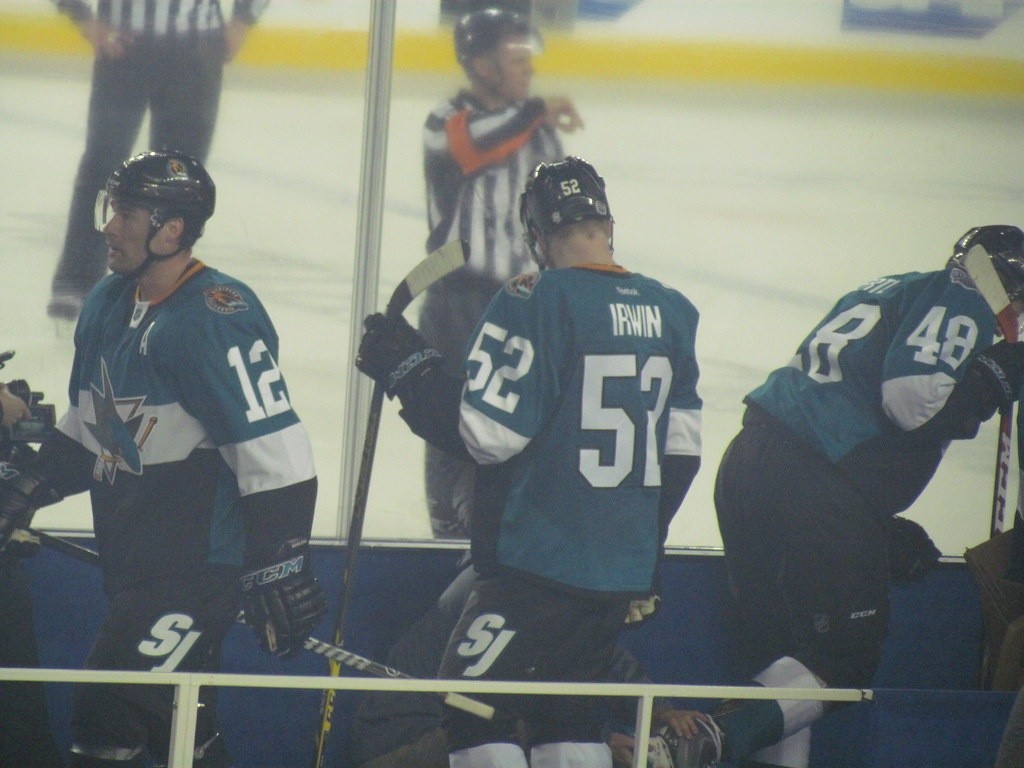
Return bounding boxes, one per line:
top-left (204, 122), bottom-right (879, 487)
top-left (431, 497), bottom-right (473, 541)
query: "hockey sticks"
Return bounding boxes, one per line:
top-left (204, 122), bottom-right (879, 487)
top-left (0, 523), bottom-right (517, 730)
top-left (963, 242), bottom-right (1020, 688)
top-left (311, 238), bottom-right (472, 767)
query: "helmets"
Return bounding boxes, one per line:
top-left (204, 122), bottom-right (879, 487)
top-left (105, 151), bottom-right (215, 229)
top-left (519, 155), bottom-right (614, 270)
top-left (454, 8), bottom-right (538, 63)
top-left (945, 224), bottom-right (1024, 303)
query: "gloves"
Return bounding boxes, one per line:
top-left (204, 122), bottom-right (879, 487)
top-left (0, 443), bottom-right (64, 546)
top-left (623, 516), bottom-right (669, 627)
top-left (964, 338), bottom-right (1024, 421)
top-left (234, 534), bottom-right (325, 656)
top-left (886, 515), bottom-right (940, 584)
top-left (355, 303), bottom-right (442, 407)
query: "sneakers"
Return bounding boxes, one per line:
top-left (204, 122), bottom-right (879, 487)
top-left (631, 714), bottom-right (729, 768)
top-left (46, 265), bottom-right (92, 344)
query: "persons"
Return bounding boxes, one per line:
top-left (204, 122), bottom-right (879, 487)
top-left (355, 155), bottom-right (1024, 768)
top-left (46, 0), bottom-right (271, 320)
top-left (1, 150), bottom-right (330, 768)
top-left (420, 8), bottom-right (587, 539)
